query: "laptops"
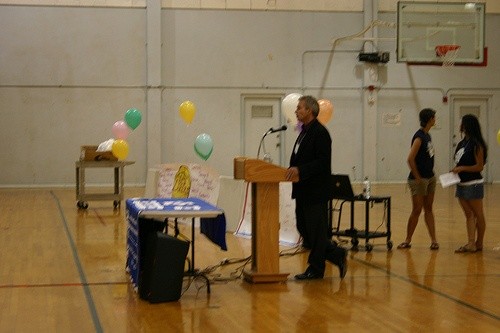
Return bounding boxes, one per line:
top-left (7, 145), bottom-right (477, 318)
top-left (332, 175), bottom-right (358, 199)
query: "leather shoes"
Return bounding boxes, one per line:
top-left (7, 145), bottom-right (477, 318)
top-left (295, 269), bottom-right (325, 280)
top-left (337, 248), bottom-right (348, 278)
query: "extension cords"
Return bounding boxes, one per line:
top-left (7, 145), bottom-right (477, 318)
top-left (223, 258), bottom-right (229, 264)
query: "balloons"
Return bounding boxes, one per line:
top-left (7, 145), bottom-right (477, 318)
top-left (193, 133), bottom-right (213, 160)
top-left (112, 107), bottom-right (142, 161)
top-left (178, 101), bottom-right (196, 125)
top-left (281, 93), bottom-right (333, 129)
top-left (496, 130), bottom-right (500, 145)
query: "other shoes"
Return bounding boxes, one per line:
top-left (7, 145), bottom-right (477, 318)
top-left (455, 243), bottom-right (484, 254)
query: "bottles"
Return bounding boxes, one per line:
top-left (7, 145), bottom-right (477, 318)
top-left (362, 176), bottom-right (371, 199)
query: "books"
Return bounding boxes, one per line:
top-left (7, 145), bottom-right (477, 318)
top-left (439, 172), bottom-right (461, 188)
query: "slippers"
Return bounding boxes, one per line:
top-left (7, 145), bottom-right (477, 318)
top-left (397, 243), bottom-right (412, 249)
top-left (431, 242), bottom-right (439, 249)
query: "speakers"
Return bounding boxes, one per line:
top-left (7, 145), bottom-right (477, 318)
top-left (139, 231), bottom-right (189, 304)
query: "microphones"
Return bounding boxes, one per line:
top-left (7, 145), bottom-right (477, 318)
top-left (257, 125), bottom-right (287, 158)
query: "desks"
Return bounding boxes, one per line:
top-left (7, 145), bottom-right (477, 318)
top-left (125, 198), bottom-right (228, 295)
top-left (75, 160), bottom-right (135, 209)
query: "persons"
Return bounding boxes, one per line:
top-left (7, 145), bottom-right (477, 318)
top-left (397, 108), bottom-right (440, 250)
top-left (448, 114), bottom-right (489, 253)
top-left (285, 95), bottom-right (348, 280)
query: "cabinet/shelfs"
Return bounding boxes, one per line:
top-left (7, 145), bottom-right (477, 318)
top-left (330, 193), bottom-right (394, 253)
